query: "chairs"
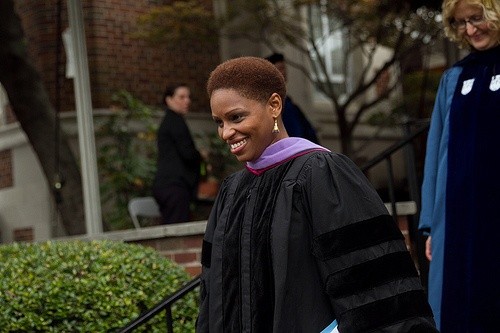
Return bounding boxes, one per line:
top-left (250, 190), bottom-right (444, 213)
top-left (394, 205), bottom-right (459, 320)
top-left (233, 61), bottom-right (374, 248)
top-left (128, 196), bottom-right (161, 229)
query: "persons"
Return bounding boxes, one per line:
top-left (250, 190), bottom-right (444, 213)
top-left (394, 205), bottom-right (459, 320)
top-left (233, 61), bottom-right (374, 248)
top-left (194, 56), bottom-right (438, 333)
top-left (418, 0), bottom-right (500, 333)
top-left (265, 50), bottom-right (322, 147)
top-left (152, 82), bottom-right (213, 227)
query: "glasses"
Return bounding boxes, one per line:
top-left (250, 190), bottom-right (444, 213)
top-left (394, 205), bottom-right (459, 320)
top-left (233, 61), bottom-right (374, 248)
top-left (451, 15), bottom-right (484, 30)
